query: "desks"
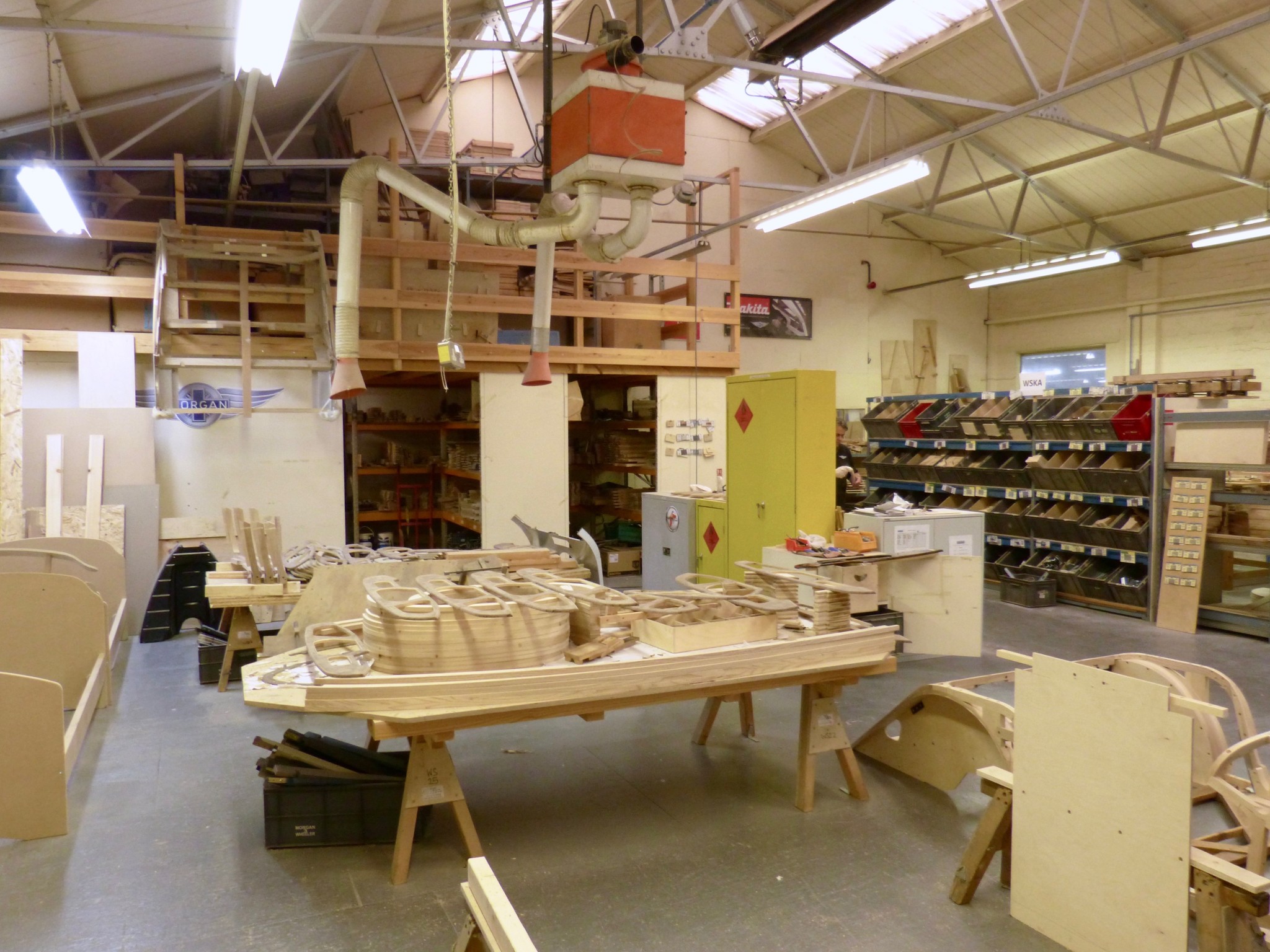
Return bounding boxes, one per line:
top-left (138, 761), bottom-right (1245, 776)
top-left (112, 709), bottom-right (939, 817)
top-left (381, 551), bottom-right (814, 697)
top-left (242, 632), bottom-right (907, 890)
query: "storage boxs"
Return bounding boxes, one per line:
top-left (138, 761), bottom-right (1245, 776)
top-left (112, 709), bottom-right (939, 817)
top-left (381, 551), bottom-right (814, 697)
top-left (258, 752), bottom-right (434, 847)
top-left (856, 397), bottom-right (1158, 612)
top-left (193, 640), bottom-right (259, 684)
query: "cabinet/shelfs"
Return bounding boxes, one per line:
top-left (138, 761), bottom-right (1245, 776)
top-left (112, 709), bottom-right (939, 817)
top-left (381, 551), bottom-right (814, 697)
top-left (852, 391), bottom-right (1168, 621)
top-left (560, 385), bottom-right (672, 579)
top-left (338, 401), bottom-right (491, 556)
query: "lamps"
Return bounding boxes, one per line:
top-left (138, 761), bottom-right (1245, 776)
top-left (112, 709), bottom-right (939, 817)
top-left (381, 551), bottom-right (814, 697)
top-left (14, 164), bottom-right (99, 244)
top-left (747, 155), bottom-right (932, 237)
top-left (231, 0), bottom-right (303, 92)
top-left (961, 247), bottom-right (1122, 290)
top-left (1188, 213), bottom-right (1269, 251)
